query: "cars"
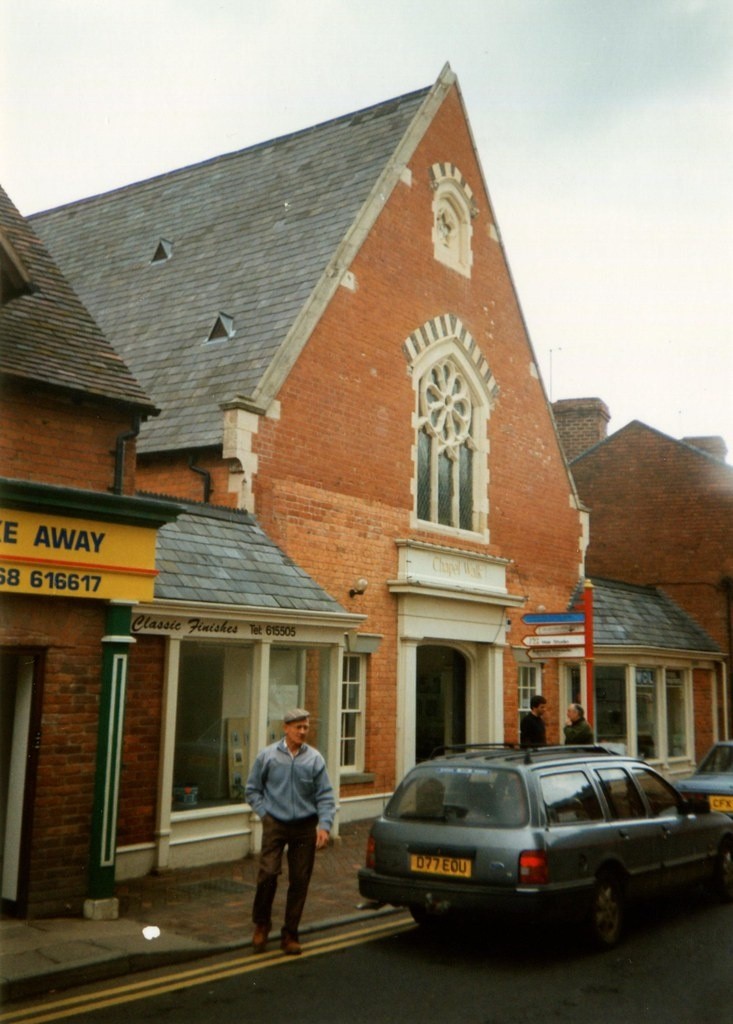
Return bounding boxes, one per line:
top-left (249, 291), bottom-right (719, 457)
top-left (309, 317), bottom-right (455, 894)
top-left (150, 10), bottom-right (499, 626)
top-left (668, 741), bottom-right (733, 821)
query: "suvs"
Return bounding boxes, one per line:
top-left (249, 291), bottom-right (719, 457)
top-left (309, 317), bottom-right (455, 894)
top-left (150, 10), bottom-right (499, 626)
top-left (357, 744), bottom-right (732, 949)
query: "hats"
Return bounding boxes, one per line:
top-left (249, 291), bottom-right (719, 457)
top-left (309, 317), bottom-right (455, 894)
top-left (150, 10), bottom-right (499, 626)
top-left (284, 708), bottom-right (309, 723)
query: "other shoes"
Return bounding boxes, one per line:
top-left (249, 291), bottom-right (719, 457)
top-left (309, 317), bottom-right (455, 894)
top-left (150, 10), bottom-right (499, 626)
top-left (280, 932), bottom-right (301, 955)
top-left (252, 923), bottom-right (272, 950)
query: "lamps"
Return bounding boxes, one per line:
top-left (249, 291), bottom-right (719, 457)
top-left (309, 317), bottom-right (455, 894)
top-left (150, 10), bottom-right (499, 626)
top-left (535, 605), bottom-right (547, 614)
top-left (349, 578), bottom-right (368, 596)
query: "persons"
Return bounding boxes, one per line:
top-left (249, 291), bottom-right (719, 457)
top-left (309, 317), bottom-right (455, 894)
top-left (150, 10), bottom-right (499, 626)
top-left (520, 695), bottom-right (547, 751)
top-left (244, 709), bottom-right (335, 957)
top-left (563, 703), bottom-right (595, 745)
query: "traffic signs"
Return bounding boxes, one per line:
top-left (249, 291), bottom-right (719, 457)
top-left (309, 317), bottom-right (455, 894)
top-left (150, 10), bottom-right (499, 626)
top-left (521, 635), bottom-right (586, 647)
top-left (533, 625), bottom-right (585, 636)
top-left (520, 612), bottom-right (585, 624)
top-left (526, 647), bottom-right (586, 659)
